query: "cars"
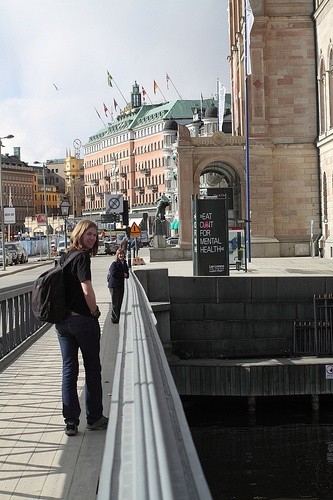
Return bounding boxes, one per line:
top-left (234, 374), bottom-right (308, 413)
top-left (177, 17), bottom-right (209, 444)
top-left (46, 240), bottom-right (72, 257)
top-left (97, 230), bottom-right (149, 255)
top-left (0, 242), bottom-right (28, 266)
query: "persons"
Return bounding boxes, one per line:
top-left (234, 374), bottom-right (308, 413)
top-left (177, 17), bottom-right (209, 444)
top-left (107, 249), bottom-right (129, 324)
top-left (56, 219), bottom-right (109, 437)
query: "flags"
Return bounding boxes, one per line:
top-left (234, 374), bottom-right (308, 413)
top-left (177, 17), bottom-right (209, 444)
top-left (167, 75), bottom-right (169, 88)
top-left (114, 99), bottom-right (118, 110)
top-left (142, 88), bottom-right (146, 96)
top-left (104, 103), bottom-right (108, 114)
top-left (108, 73), bottom-right (113, 87)
top-left (154, 82), bottom-right (158, 94)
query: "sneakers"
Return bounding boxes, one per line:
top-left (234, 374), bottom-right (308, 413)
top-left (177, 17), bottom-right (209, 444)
top-left (65, 419), bottom-right (80, 436)
top-left (87, 415), bottom-right (109, 431)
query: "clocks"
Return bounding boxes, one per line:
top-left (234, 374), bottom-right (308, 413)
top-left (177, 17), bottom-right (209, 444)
top-left (73, 138), bottom-right (82, 150)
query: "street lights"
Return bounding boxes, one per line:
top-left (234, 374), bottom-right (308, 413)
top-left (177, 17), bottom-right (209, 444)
top-left (0, 134), bottom-right (15, 271)
top-left (33, 160), bottom-right (52, 260)
top-left (59, 196), bottom-right (70, 249)
top-left (83, 184), bottom-right (92, 222)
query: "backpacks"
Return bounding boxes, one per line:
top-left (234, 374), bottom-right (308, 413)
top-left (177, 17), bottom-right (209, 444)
top-left (32, 251), bottom-right (87, 324)
